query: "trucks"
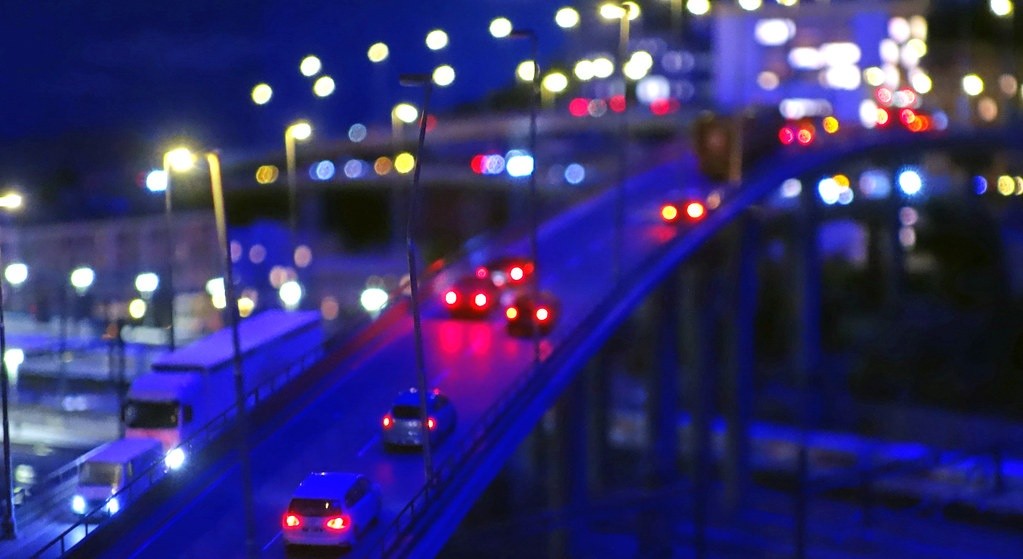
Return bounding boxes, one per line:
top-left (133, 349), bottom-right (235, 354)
top-left (74, 440), bottom-right (166, 521)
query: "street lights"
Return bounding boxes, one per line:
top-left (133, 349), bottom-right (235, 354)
top-left (173, 149), bottom-right (261, 559)
top-left (286, 123), bottom-right (310, 306)
top-left (502, 27), bottom-right (539, 363)
top-left (395, 71), bottom-right (433, 488)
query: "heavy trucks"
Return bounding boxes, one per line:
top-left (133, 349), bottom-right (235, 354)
top-left (120, 309), bottom-right (327, 469)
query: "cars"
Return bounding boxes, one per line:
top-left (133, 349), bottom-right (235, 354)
top-left (443, 258), bottom-right (535, 317)
top-left (508, 293), bottom-right (553, 337)
top-left (383, 390), bottom-right (455, 454)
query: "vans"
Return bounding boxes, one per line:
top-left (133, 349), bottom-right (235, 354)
top-left (281, 471), bottom-right (381, 552)
